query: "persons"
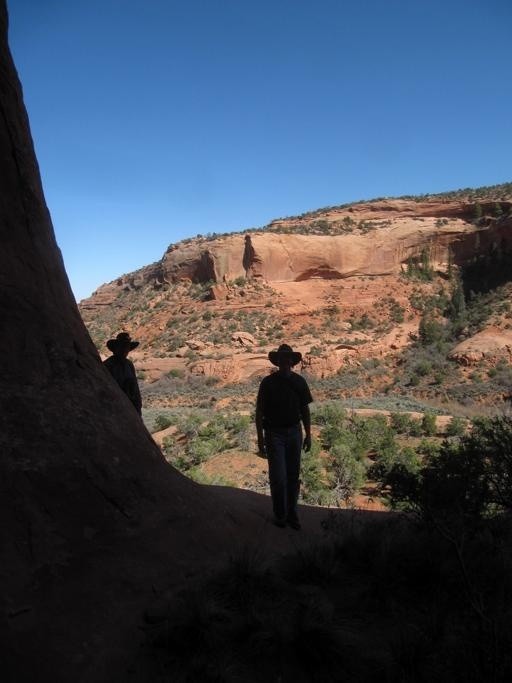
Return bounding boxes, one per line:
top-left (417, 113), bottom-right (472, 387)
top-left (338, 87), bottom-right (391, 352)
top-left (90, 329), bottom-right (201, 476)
top-left (103, 332), bottom-right (142, 419)
top-left (256, 344), bottom-right (314, 531)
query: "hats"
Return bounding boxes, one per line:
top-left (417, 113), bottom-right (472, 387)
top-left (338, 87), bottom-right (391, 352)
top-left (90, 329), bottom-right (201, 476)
top-left (268, 345), bottom-right (302, 367)
top-left (107, 333), bottom-right (139, 352)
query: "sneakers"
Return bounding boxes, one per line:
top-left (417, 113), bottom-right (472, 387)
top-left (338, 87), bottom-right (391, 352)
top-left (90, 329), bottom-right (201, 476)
top-left (275, 515), bottom-right (301, 531)
